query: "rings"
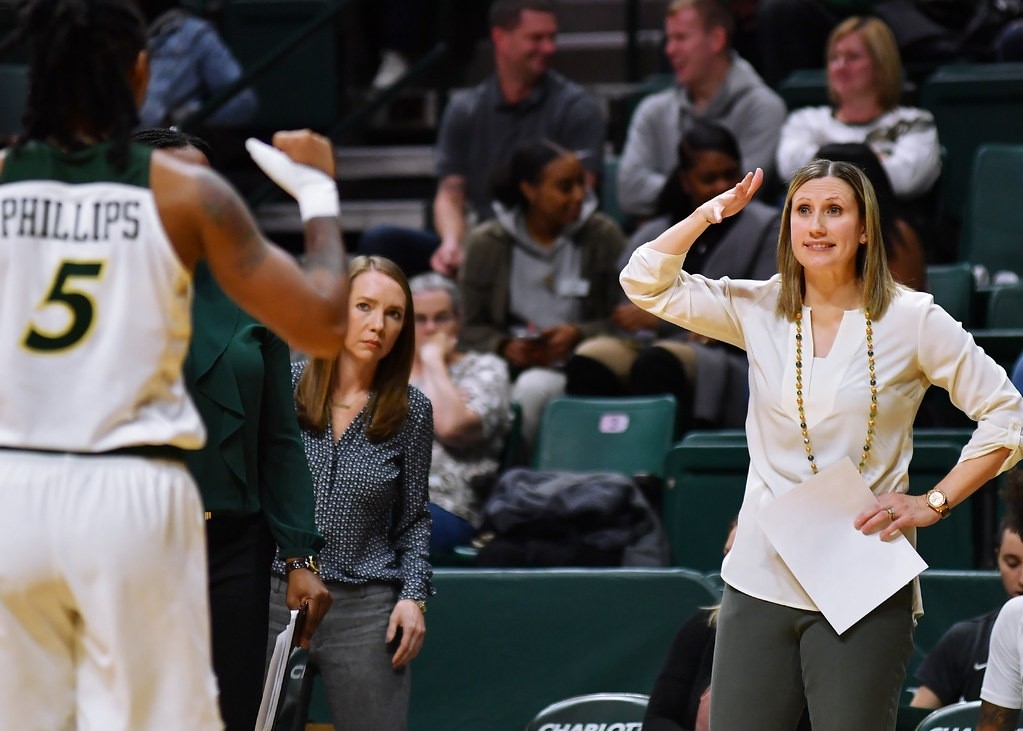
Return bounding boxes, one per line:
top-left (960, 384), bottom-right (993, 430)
top-left (887, 508), bottom-right (895, 520)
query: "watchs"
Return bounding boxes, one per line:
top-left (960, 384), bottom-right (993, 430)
top-left (926, 486), bottom-right (952, 520)
top-left (412, 600), bottom-right (428, 614)
top-left (283, 554), bottom-right (322, 576)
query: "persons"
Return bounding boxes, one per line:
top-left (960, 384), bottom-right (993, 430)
top-left (0, 0), bottom-right (350, 730)
top-left (268, 255), bottom-right (435, 731)
top-left (618, 159), bottom-right (1023, 731)
top-left (136, 123), bottom-right (335, 731)
top-left (124, 0), bottom-right (948, 565)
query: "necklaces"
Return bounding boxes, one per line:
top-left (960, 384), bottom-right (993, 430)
top-left (794, 288), bottom-right (878, 477)
top-left (332, 391), bottom-right (363, 410)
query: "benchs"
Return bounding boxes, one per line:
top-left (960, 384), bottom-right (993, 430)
top-left (241, 0), bottom-right (1023, 731)
